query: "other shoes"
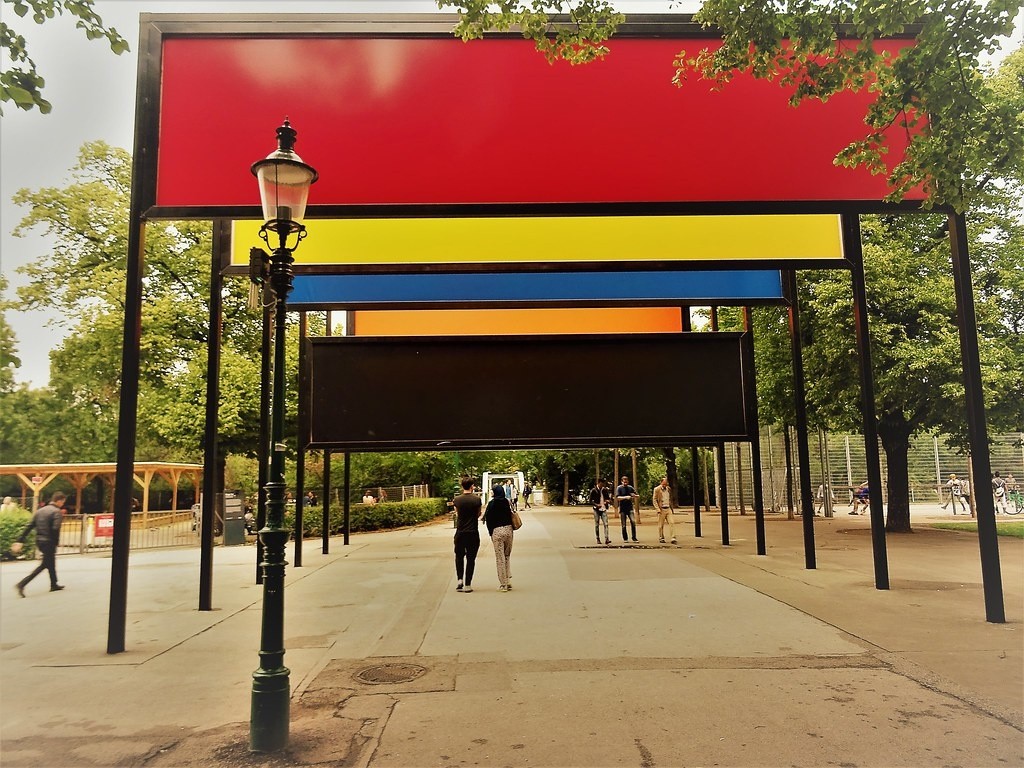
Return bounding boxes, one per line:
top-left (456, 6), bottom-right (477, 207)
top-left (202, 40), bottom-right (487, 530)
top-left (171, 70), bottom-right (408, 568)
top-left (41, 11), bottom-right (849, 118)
top-left (465, 587), bottom-right (473, 593)
top-left (671, 540), bottom-right (677, 543)
top-left (500, 585), bottom-right (506, 592)
top-left (507, 585), bottom-right (512, 590)
top-left (624, 539), bottom-right (628, 543)
top-left (596, 540), bottom-right (600, 544)
top-left (632, 539), bottom-right (640, 543)
top-left (456, 582), bottom-right (463, 590)
top-left (605, 540), bottom-right (611, 544)
top-left (16, 584), bottom-right (26, 598)
top-left (660, 540), bottom-right (666, 543)
top-left (50, 585), bottom-right (65, 592)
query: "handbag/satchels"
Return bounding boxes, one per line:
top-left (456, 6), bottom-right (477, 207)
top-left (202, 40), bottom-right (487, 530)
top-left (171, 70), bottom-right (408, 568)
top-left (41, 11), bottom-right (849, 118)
top-left (506, 497), bottom-right (522, 530)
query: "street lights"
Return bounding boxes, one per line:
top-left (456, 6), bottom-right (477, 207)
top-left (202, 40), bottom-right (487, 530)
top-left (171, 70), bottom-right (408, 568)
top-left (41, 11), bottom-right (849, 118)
top-left (249, 114), bottom-right (319, 757)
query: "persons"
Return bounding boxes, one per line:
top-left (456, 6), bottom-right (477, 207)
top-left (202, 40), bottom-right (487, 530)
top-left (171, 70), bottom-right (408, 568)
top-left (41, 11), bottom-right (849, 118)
top-left (12, 490), bottom-right (65, 598)
top-left (284, 489), bottom-right (388, 507)
top-left (817, 480), bottom-right (836, 513)
top-left (0, 495), bottom-right (15, 510)
top-left (481, 484), bottom-right (514, 592)
top-left (500, 480), bottom-right (532, 513)
top-left (941, 471), bottom-right (1020, 516)
top-left (589, 478), bottom-right (613, 544)
top-left (453, 477), bottom-right (483, 593)
top-left (848, 488), bottom-right (870, 515)
top-left (615, 476), bottom-right (640, 543)
top-left (653, 478), bottom-right (678, 544)
